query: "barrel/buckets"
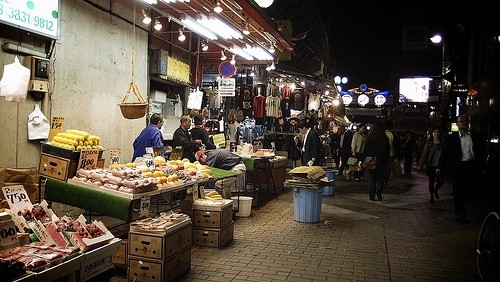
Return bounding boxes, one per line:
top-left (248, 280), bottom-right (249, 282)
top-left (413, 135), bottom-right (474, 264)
top-left (230, 196), bottom-right (253, 218)
top-left (292, 187), bottom-right (325, 223)
top-left (322, 169), bottom-right (338, 195)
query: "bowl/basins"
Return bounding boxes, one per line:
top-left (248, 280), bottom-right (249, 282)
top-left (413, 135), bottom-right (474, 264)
top-left (118, 102), bottom-right (148, 119)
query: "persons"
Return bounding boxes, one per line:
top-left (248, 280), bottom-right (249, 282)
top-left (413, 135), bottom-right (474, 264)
top-left (436, 117), bottom-right (486, 223)
top-left (295, 120), bottom-right (325, 170)
top-left (364, 120), bottom-right (391, 201)
top-left (331, 123), bottom-right (416, 181)
top-left (132, 113), bottom-right (164, 163)
top-left (172, 115), bottom-right (221, 161)
top-left (417, 128), bottom-right (449, 201)
top-left (195, 149), bottom-right (246, 199)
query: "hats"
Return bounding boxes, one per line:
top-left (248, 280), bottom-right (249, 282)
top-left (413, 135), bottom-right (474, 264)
top-left (195, 149), bottom-right (205, 161)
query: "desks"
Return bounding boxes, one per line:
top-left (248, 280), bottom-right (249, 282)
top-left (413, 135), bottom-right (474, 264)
top-left (39, 150), bottom-right (288, 235)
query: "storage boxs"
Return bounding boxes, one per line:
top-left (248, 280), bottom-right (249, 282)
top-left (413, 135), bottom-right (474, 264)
top-left (112, 199), bottom-right (234, 281)
top-left (0, 150), bottom-right (105, 204)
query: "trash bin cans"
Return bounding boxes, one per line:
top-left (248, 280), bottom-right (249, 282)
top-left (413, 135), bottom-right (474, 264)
top-left (292, 174), bottom-right (324, 224)
top-left (322, 169), bottom-right (337, 196)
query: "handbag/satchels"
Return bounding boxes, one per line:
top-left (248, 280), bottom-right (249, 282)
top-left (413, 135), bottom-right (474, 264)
top-left (364, 156), bottom-right (376, 169)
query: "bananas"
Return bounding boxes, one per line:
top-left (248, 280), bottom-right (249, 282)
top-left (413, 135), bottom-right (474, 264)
top-left (205, 191), bottom-right (224, 200)
top-left (168, 158), bottom-right (212, 178)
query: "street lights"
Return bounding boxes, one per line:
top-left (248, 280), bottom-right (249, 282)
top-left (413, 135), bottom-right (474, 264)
top-left (429, 35), bottom-right (445, 106)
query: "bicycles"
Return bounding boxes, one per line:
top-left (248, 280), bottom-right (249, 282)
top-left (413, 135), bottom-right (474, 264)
top-left (475, 212), bottom-right (500, 282)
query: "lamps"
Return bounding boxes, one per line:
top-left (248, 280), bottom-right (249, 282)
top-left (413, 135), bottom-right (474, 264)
top-left (141, 0), bottom-right (276, 71)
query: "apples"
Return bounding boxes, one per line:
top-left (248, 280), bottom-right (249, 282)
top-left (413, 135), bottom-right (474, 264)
top-left (123, 162), bottom-right (197, 188)
top-left (50, 129), bottom-right (103, 152)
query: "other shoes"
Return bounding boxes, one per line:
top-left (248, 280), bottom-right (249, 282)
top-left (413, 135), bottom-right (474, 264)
top-left (376, 191), bottom-right (382, 201)
top-left (434, 191), bottom-right (439, 200)
top-left (430, 198), bottom-right (434, 202)
